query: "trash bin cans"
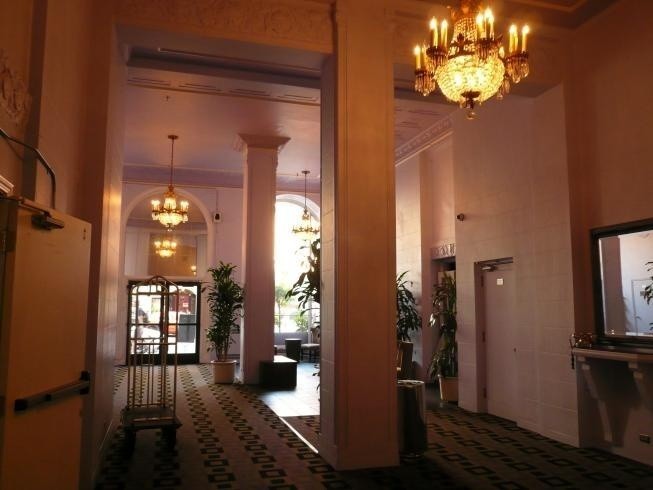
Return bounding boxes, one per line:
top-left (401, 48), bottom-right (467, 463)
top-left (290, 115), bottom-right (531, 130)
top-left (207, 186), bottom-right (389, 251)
top-left (397, 380), bottom-right (428, 458)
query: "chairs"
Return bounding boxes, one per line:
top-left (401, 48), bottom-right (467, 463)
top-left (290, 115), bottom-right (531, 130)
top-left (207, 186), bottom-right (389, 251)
top-left (301, 325), bottom-right (321, 362)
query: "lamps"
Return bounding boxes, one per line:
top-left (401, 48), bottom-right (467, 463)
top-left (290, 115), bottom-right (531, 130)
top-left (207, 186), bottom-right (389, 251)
top-left (150, 135), bottom-right (189, 232)
top-left (414, 0), bottom-right (530, 119)
top-left (292, 171), bottom-right (320, 241)
top-left (154, 234), bottom-right (176, 258)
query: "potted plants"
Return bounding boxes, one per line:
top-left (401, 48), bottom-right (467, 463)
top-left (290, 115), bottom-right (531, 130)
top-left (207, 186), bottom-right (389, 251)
top-left (201, 260), bottom-right (246, 383)
top-left (427, 271), bottom-right (458, 402)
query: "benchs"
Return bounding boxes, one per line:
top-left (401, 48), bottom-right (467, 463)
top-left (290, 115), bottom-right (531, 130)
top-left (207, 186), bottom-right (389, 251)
top-left (274, 332), bottom-right (308, 355)
top-left (260, 355), bottom-right (297, 389)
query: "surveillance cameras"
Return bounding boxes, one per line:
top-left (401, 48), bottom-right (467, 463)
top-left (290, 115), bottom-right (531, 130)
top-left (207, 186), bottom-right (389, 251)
top-left (456, 214), bottom-right (464, 222)
